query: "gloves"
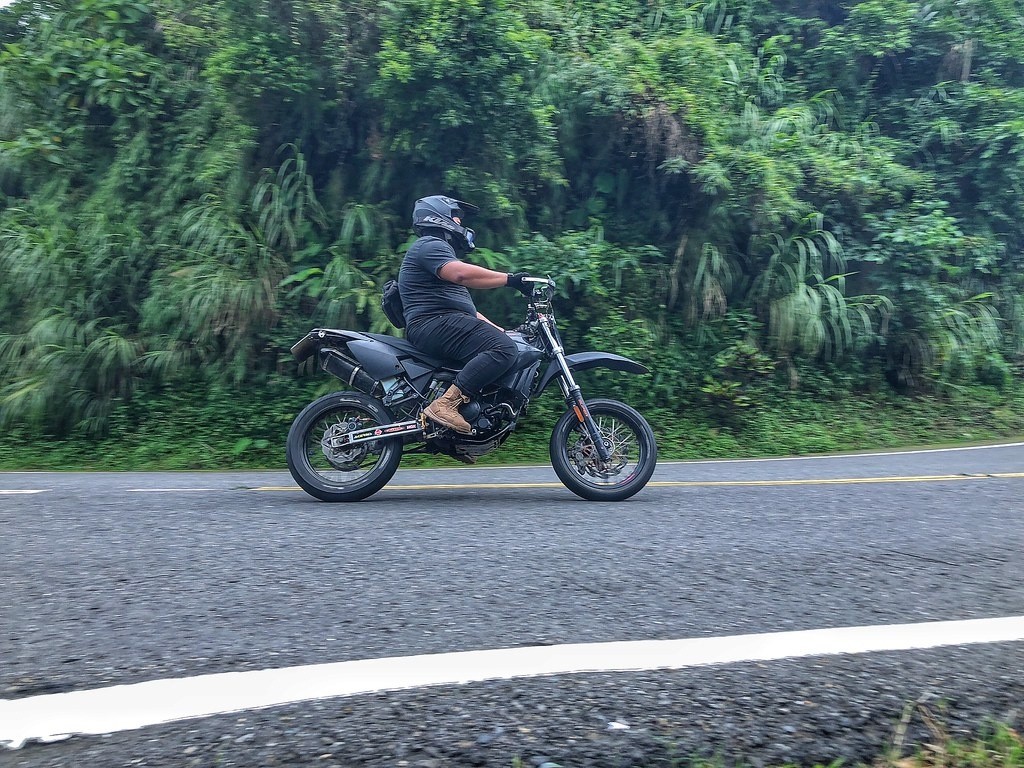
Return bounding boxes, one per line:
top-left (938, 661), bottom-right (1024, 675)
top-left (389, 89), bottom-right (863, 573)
top-left (507, 272), bottom-right (535, 296)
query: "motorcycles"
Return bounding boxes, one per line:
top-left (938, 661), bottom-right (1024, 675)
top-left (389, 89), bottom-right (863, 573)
top-left (285, 274), bottom-right (658, 503)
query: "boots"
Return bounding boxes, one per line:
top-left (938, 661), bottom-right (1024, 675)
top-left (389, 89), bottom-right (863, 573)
top-left (449, 450), bottom-right (476, 464)
top-left (424, 384), bottom-right (471, 435)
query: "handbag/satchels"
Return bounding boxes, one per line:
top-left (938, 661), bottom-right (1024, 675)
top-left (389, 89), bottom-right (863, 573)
top-left (381, 280), bottom-right (406, 328)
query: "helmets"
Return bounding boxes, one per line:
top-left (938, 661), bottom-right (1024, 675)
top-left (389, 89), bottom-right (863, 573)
top-left (412, 195), bottom-right (480, 258)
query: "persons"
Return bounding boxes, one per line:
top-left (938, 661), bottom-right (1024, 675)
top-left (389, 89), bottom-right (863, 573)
top-left (399, 196), bottom-right (535, 463)
